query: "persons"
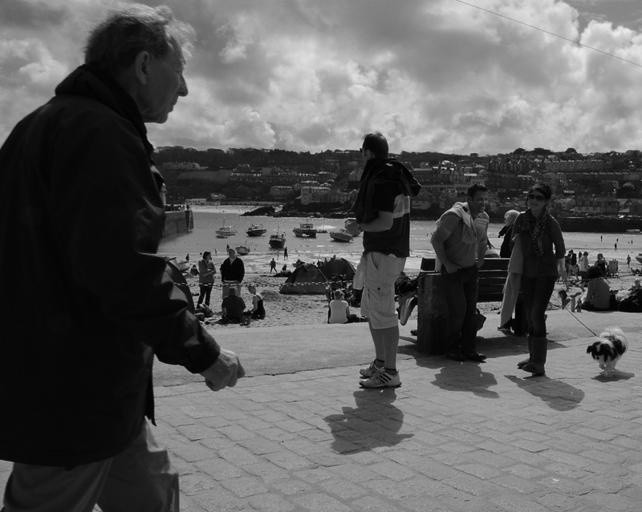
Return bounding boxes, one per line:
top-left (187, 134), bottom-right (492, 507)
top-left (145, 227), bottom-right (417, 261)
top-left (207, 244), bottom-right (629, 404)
top-left (352, 131), bottom-right (421, 389)
top-left (565, 248), bottom-right (610, 311)
top-left (178, 242), bottom-right (287, 322)
top-left (498, 209), bottom-right (520, 258)
top-left (327, 289), bottom-right (358, 323)
top-left (512, 185), bottom-right (565, 372)
top-left (0, 6), bottom-right (246, 512)
top-left (431, 183), bottom-right (489, 362)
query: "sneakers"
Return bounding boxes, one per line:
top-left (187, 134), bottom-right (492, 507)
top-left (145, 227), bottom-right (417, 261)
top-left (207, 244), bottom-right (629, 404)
top-left (359, 367), bottom-right (401, 389)
top-left (359, 361), bottom-right (378, 377)
top-left (456, 351), bottom-right (486, 361)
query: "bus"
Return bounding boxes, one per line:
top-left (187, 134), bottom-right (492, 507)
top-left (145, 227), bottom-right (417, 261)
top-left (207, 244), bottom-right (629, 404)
top-left (569, 197), bottom-right (619, 217)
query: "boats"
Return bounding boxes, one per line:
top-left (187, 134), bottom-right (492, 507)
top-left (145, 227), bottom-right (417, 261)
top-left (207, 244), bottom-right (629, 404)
top-left (268, 225), bottom-right (286, 249)
top-left (293, 222), bottom-right (317, 238)
top-left (246, 223), bottom-right (267, 236)
top-left (234, 246), bottom-right (251, 256)
top-left (216, 226), bottom-right (236, 238)
top-left (341, 217), bottom-right (363, 237)
top-left (329, 230), bottom-right (352, 242)
top-left (317, 229), bottom-right (328, 234)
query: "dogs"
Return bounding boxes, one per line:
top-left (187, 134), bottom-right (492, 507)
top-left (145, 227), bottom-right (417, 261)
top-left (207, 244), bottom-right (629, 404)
top-left (585, 326), bottom-right (628, 377)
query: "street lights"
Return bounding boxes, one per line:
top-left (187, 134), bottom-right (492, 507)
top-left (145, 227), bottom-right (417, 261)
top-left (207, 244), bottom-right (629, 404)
top-left (612, 189), bottom-right (618, 217)
top-left (591, 193), bottom-right (596, 216)
